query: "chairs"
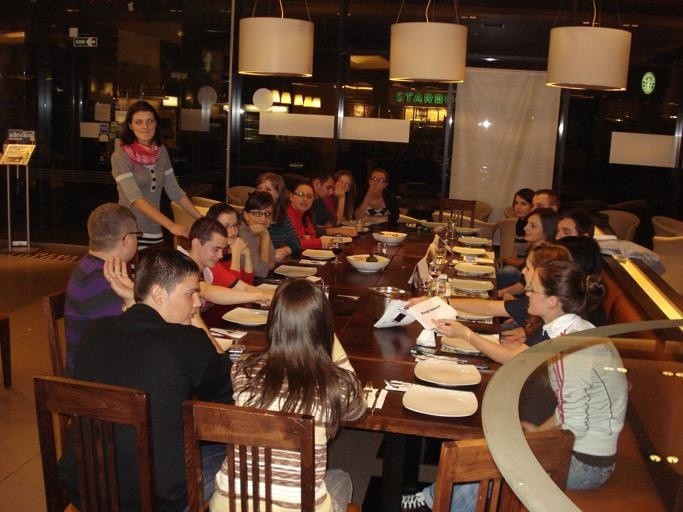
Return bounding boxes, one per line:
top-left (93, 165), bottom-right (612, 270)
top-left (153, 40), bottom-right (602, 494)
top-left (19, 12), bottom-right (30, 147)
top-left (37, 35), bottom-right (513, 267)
top-left (33, 186), bottom-right (682, 512)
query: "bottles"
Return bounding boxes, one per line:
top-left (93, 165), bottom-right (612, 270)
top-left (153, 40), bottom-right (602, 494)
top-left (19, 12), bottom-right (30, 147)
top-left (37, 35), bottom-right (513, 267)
top-left (377, 243), bottom-right (387, 257)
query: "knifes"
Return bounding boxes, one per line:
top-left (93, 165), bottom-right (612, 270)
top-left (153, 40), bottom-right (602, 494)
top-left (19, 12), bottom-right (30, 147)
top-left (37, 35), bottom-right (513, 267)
top-left (371, 389), bottom-right (382, 415)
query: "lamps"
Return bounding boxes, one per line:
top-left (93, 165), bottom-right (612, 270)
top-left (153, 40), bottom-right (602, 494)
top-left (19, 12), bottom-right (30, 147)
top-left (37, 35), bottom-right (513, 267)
top-left (238, 0), bottom-right (315, 78)
top-left (271, 90), bottom-right (322, 108)
top-left (389, 0), bottom-right (468, 83)
top-left (545, 0), bottom-right (632, 91)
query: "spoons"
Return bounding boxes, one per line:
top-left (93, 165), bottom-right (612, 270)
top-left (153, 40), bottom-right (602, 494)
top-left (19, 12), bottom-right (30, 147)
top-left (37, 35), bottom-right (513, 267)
top-left (366, 250), bottom-right (377, 262)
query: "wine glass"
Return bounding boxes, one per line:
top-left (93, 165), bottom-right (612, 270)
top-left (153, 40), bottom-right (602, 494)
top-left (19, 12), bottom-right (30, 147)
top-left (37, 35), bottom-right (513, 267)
top-left (331, 235), bottom-right (344, 265)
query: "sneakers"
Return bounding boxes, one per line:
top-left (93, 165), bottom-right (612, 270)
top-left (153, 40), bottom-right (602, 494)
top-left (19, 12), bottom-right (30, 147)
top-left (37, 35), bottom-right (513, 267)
top-left (346, 503), bottom-right (361, 512)
top-left (401, 493), bottom-right (432, 512)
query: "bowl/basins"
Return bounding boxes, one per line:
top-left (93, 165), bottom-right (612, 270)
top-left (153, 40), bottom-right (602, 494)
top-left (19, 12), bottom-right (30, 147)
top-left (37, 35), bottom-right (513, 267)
top-left (346, 253), bottom-right (391, 273)
top-left (372, 230), bottom-right (409, 246)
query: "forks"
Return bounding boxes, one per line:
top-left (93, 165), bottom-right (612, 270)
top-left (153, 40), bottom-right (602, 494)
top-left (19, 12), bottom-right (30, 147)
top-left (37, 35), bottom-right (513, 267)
top-left (364, 380), bottom-right (373, 397)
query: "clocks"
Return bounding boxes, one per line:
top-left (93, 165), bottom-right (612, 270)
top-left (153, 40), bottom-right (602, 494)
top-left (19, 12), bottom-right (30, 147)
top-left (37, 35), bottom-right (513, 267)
top-left (639, 71), bottom-right (665, 104)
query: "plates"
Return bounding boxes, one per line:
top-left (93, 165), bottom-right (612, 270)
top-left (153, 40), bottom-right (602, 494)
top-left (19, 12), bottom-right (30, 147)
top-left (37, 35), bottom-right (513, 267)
top-left (221, 306), bottom-right (268, 328)
top-left (453, 227), bottom-right (489, 256)
top-left (274, 265), bottom-right (318, 278)
top-left (402, 360), bottom-right (482, 417)
top-left (450, 263), bottom-right (496, 293)
top-left (320, 234), bottom-right (352, 244)
top-left (303, 249), bottom-right (333, 260)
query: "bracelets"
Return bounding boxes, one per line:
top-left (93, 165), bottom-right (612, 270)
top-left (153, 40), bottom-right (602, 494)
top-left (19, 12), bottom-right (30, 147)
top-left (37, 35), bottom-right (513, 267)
top-left (463, 330), bottom-right (474, 343)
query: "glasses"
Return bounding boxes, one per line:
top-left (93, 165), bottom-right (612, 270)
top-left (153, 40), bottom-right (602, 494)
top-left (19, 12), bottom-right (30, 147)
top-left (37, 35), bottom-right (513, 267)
top-left (369, 175), bottom-right (386, 184)
top-left (524, 284), bottom-right (549, 296)
top-left (291, 191), bottom-right (313, 201)
top-left (247, 208), bottom-right (274, 218)
top-left (122, 230), bottom-right (145, 240)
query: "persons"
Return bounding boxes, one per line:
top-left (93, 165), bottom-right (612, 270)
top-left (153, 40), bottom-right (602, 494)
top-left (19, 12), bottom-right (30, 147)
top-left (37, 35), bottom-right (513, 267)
top-left (203, 203), bottom-right (255, 312)
top-left (322, 170), bottom-right (357, 227)
top-left (237, 191), bottom-right (292, 279)
top-left (353, 168), bottom-right (400, 226)
top-left (210, 278), bottom-right (366, 510)
top-left (256, 173), bottom-right (300, 263)
top-left (111, 100), bottom-right (203, 278)
top-left (309, 170), bottom-right (358, 237)
top-left (404, 241), bottom-right (573, 427)
top-left (497, 190), bottom-right (560, 290)
top-left (499, 207), bottom-right (608, 345)
top-left (64, 203), bottom-right (143, 378)
top-left (497, 208), bottom-right (560, 300)
top-left (498, 190), bottom-right (536, 271)
top-left (396, 261), bottom-right (627, 509)
top-left (284, 180), bottom-right (333, 251)
top-left (176, 217), bottom-right (275, 314)
top-left (67, 249), bottom-right (235, 510)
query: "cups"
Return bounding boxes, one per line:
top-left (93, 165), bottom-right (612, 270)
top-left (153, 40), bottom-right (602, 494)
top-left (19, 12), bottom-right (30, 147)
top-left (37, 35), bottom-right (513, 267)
top-left (356, 220), bottom-right (364, 236)
top-left (428, 262), bottom-right (446, 298)
